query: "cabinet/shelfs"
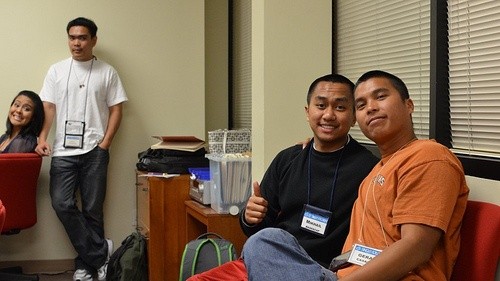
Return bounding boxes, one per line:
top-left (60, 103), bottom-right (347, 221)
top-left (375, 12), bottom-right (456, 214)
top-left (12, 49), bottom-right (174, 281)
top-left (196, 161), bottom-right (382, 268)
top-left (134, 168), bottom-right (192, 281)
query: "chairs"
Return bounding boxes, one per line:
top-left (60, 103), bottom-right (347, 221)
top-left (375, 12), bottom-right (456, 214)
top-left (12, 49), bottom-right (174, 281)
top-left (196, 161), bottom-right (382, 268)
top-left (0, 152), bottom-right (42, 281)
top-left (450, 199), bottom-right (500, 281)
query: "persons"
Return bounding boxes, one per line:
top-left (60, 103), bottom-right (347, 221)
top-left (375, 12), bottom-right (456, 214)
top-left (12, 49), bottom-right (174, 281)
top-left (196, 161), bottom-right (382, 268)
top-left (1, 89), bottom-right (45, 156)
top-left (34, 17), bottom-right (129, 281)
top-left (240, 70), bottom-right (470, 281)
top-left (184, 72), bottom-right (382, 281)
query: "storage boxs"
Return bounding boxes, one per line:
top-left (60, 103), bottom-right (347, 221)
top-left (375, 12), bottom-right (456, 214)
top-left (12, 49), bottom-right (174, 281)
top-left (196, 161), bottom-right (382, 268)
top-left (188, 154), bottom-right (252, 214)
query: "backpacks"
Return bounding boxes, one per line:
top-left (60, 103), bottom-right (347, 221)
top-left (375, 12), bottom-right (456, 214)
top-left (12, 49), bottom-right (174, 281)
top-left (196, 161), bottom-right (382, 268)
top-left (106, 232), bottom-right (149, 281)
top-left (179, 232), bottom-right (238, 281)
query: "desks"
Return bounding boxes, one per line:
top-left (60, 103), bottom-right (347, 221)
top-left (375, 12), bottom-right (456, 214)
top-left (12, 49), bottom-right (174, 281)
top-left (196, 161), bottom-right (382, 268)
top-left (183, 200), bottom-right (248, 260)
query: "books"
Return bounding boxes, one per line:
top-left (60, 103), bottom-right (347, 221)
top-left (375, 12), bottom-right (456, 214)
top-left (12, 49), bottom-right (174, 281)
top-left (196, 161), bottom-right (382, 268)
top-left (151, 135), bottom-right (206, 153)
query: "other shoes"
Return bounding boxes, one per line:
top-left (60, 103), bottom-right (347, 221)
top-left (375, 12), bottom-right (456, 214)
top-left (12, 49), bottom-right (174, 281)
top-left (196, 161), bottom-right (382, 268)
top-left (96, 238), bottom-right (114, 281)
top-left (72, 269), bottom-right (93, 281)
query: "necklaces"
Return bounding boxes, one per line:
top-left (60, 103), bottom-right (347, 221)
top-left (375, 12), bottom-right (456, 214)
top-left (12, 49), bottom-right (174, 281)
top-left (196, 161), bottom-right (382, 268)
top-left (72, 58), bottom-right (94, 89)
